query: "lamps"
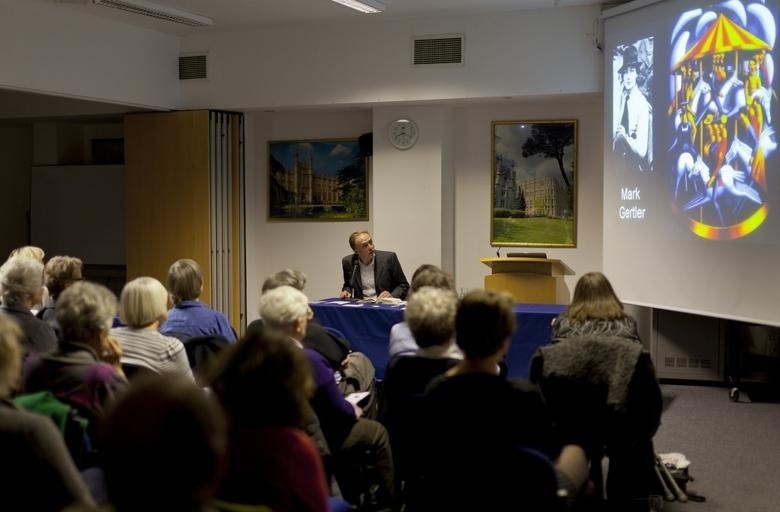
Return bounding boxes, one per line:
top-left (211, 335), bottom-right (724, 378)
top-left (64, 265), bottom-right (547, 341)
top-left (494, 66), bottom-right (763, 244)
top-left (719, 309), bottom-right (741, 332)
top-left (330, 0), bottom-right (387, 14)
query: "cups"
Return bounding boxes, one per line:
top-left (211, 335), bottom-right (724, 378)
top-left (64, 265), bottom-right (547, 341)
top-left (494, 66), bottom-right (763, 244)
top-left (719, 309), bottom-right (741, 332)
top-left (459, 288), bottom-right (469, 300)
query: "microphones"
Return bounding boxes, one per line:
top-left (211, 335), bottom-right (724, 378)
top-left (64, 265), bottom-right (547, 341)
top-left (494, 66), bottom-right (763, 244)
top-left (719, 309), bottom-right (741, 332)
top-left (349, 259), bottom-right (359, 286)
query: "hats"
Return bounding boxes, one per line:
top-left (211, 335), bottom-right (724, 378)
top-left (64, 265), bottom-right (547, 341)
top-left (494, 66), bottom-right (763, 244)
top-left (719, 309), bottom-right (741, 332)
top-left (618, 46), bottom-right (642, 72)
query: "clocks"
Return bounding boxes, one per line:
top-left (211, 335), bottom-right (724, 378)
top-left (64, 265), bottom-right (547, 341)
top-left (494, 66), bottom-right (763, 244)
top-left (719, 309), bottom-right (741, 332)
top-left (388, 118), bottom-right (419, 151)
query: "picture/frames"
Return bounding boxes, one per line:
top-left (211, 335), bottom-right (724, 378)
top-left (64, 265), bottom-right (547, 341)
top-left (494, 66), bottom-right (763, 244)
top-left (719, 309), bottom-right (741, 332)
top-left (489, 118), bottom-right (580, 248)
top-left (265, 137), bottom-right (370, 221)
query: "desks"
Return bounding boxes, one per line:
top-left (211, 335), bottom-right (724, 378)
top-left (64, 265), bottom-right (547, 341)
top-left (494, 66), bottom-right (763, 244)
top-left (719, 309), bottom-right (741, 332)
top-left (306, 297), bottom-right (571, 384)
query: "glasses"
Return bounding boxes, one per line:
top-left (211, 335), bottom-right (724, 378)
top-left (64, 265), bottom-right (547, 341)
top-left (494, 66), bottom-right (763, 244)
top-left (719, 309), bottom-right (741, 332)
top-left (73, 276), bottom-right (86, 281)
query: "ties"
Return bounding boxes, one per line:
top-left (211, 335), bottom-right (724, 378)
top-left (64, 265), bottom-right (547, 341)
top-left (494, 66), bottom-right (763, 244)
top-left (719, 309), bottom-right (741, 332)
top-left (621, 94), bottom-right (630, 135)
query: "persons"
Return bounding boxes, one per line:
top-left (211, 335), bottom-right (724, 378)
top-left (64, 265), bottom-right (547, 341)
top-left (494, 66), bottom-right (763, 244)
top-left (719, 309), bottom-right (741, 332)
top-left (0, 312), bottom-right (97, 512)
top-left (36, 255), bottom-right (84, 316)
top-left (3, 255), bottom-right (58, 353)
top-left (111, 278), bottom-right (198, 389)
top-left (98, 381), bottom-right (222, 506)
top-left (260, 285), bottom-right (395, 499)
top-left (246, 269), bottom-right (377, 398)
top-left (15, 281), bottom-right (128, 415)
top-left (376, 287), bottom-right (502, 390)
top-left (158, 259), bottom-right (237, 343)
top-left (339, 231), bottom-right (411, 301)
top-left (7, 246), bottom-right (50, 317)
top-left (217, 332), bottom-right (329, 508)
top-left (550, 272), bottom-right (645, 340)
top-left (425, 290), bottom-right (590, 500)
top-left (388, 265), bottom-right (465, 362)
